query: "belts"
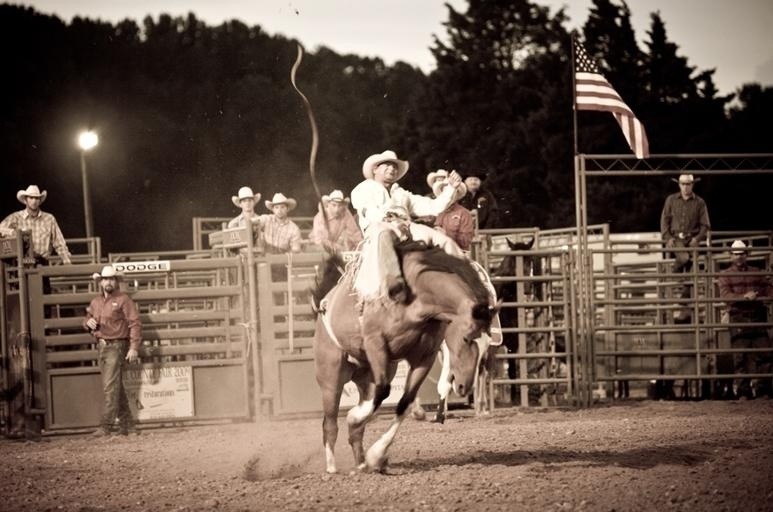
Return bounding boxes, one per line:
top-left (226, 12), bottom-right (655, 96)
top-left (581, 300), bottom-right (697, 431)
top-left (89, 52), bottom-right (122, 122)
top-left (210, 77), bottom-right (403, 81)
top-left (98, 337), bottom-right (129, 349)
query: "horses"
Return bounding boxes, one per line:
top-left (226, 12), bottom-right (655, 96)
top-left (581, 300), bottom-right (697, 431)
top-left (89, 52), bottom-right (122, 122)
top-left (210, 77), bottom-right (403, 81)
top-left (306, 222), bottom-right (504, 474)
top-left (490, 236), bottom-right (537, 406)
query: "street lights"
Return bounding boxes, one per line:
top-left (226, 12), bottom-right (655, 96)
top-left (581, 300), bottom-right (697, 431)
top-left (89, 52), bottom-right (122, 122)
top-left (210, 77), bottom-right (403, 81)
top-left (77, 130), bottom-right (98, 256)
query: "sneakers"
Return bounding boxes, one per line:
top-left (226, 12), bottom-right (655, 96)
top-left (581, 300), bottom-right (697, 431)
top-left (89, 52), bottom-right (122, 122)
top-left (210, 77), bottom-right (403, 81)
top-left (389, 276), bottom-right (408, 303)
top-left (91, 426), bottom-right (111, 437)
top-left (115, 428), bottom-right (128, 437)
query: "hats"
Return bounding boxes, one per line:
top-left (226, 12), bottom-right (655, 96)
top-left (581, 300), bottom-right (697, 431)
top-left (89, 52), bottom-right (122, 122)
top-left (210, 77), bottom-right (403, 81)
top-left (723, 240), bottom-right (753, 254)
top-left (671, 175), bottom-right (702, 184)
top-left (16, 184), bottom-right (48, 206)
top-left (231, 186), bottom-right (262, 208)
top-left (426, 169), bottom-right (467, 201)
top-left (322, 190), bottom-right (350, 208)
top-left (362, 151), bottom-right (410, 182)
top-left (264, 193), bottom-right (297, 213)
top-left (93, 265), bottom-right (126, 281)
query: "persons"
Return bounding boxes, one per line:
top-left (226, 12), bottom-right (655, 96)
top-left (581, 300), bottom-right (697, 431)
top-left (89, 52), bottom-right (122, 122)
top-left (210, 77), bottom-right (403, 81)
top-left (228, 185), bottom-right (261, 254)
top-left (660, 174), bottom-right (711, 306)
top-left (253, 194), bottom-right (302, 338)
top-left (717, 240), bottom-right (767, 398)
top-left (82, 266), bottom-right (142, 438)
top-left (351, 150), bottom-right (499, 303)
top-left (0, 185), bottom-right (71, 335)
top-left (309, 189), bottom-right (362, 254)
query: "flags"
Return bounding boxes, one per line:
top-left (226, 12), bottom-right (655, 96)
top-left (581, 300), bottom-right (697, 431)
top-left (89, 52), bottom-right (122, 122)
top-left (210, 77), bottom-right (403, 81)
top-left (573, 39), bottom-right (650, 159)
